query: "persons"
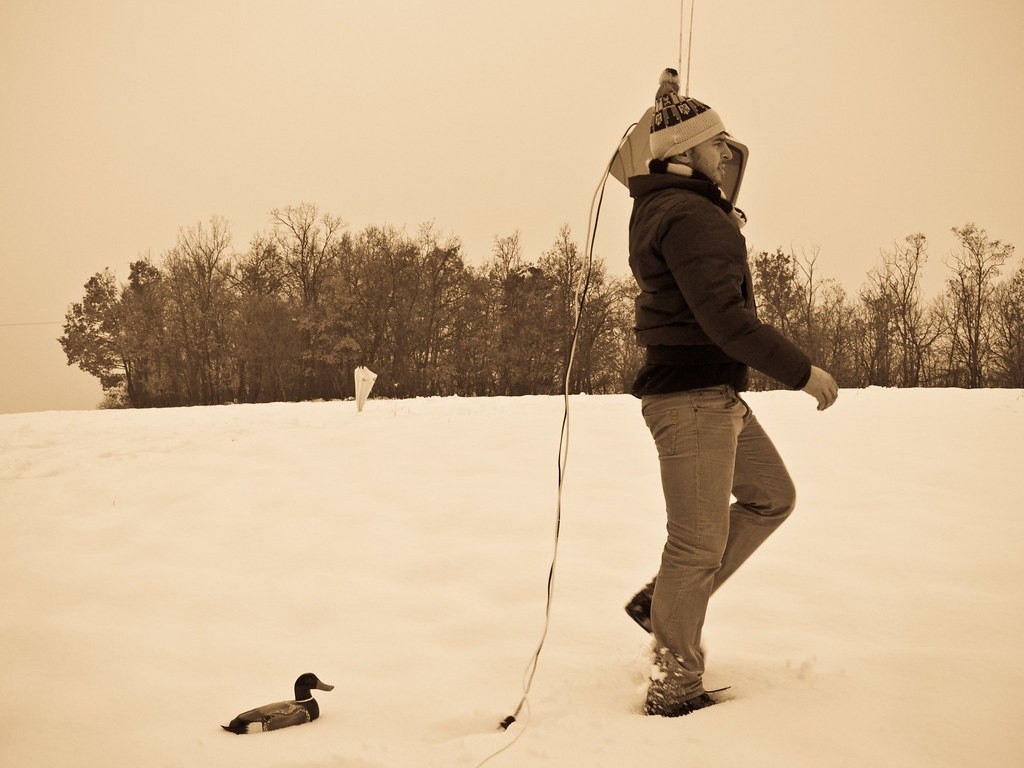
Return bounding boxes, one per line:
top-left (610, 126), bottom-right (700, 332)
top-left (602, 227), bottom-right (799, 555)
top-left (625, 67), bottom-right (841, 718)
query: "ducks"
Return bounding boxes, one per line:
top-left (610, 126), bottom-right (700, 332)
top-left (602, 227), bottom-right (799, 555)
top-left (218, 672), bottom-right (335, 735)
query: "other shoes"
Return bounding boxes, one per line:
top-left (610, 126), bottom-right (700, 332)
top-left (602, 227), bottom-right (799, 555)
top-left (627, 592), bottom-right (656, 636)
top-left (645, 686), bottom-right (731, 718)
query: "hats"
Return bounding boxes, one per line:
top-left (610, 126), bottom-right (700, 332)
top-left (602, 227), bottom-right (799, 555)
top-left (649, 68), bottom-right (725, 161)
